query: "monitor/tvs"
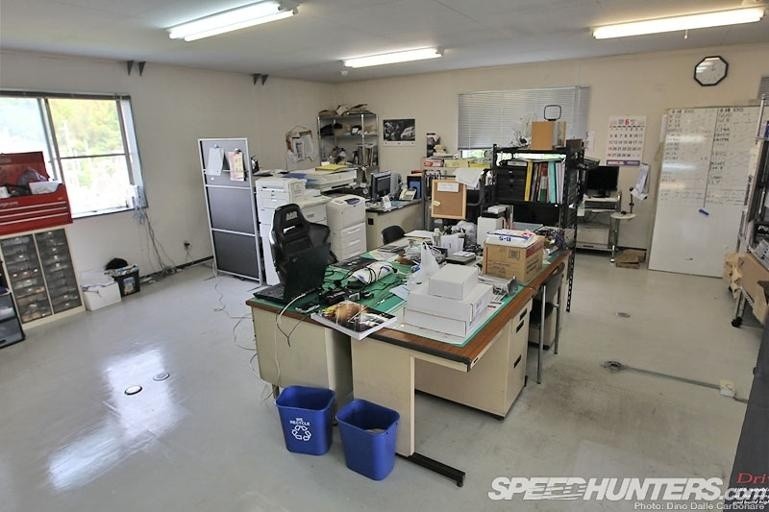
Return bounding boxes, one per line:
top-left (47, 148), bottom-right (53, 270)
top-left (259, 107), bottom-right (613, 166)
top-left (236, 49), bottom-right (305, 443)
top-left (365, 170), bottom-right (392, 203)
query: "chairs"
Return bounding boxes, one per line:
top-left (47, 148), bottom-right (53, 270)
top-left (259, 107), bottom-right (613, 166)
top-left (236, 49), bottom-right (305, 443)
top-left (269, 204), bottom-right (338, 283)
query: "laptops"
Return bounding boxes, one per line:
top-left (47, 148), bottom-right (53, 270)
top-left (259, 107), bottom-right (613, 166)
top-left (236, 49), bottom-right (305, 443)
top-left (254, 242), bottom-right (332, 304)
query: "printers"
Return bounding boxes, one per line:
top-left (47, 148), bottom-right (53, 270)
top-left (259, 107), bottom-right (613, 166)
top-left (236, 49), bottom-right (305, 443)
top-left (329, 194), bottom-right (367, 262)
top-left (304, 168), bottom-right (357, 193)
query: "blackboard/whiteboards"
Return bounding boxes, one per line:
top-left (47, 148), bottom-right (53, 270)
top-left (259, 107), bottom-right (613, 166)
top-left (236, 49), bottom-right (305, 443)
top-left (645, 104), bottom-right (769, 279)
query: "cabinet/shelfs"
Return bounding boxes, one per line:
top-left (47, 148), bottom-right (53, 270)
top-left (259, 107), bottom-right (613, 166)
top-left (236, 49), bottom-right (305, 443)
top-left (733, 123), bottom-right (769, 329)
top-left (493, 142), bottom-right (585, 314)
top-left (316, 112), bottom-right (378, 188)
top-left (0, 226), bottom-right (86, 331)
top-left (569, 191), bottom-right (622, 263)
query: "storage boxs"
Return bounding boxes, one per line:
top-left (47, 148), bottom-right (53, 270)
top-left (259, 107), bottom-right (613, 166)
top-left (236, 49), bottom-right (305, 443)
top-left (82, 282), bottom-right (122, 312)
top-left (752, 282), bottom-right (769, 326)
top-left (482, 229), bottom-right (544, 285)
top-left (0, 288), bottom-right (26, 350)
top-left (739, 249), bottom-right (769, 301)
top-left (114, 268), bottom-right (141, 296)
top-left (0, 151), bottom-right (73, 235)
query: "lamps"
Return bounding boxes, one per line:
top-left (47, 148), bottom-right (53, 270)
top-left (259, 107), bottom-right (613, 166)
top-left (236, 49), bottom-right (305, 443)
top-left (165, 0), bottom-right (296, 41)
top-left (342, 48), bottom-right (444, 69)
top-left (589, 4), bottom-right (767, 41)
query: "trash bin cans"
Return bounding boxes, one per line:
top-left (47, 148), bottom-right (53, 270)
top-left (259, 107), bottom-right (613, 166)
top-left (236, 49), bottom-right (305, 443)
top-left (274, 384), bottom-right (336, 457)
top-left (336, 398), bottom-right (401, 482)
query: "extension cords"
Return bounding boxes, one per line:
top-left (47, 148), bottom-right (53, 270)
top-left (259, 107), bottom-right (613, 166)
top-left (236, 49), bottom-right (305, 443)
top-left (135, 185), bottom-right (146, 206)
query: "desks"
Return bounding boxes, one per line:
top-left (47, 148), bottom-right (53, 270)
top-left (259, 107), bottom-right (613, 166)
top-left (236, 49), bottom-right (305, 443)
top-left (365, 193), bottom-right (425, 252)
top-left (245, 231), bottom-right (572, 487)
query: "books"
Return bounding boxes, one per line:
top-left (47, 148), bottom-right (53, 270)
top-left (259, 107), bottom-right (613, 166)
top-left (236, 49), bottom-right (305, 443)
top-left (311, 299), bottom-right (399, 339)
top-left (523, 160), bottom-right (565, 205)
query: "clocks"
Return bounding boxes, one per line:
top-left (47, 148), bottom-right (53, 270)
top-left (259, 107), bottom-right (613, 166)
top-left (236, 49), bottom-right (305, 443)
top-left (695, 55), bottom-right (729, 87)
